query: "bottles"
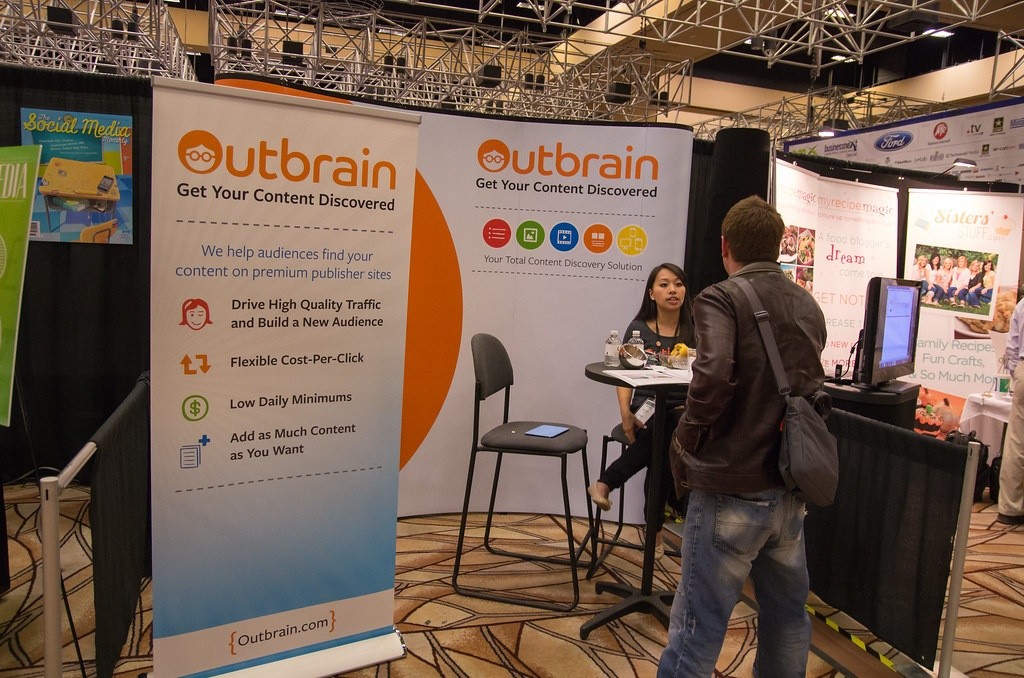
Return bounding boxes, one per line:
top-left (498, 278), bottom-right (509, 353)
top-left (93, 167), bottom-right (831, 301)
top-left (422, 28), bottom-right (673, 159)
top-left (629, 330), bottom-right (645, 352)
top-left (604, 329), bottom-right (622, 368)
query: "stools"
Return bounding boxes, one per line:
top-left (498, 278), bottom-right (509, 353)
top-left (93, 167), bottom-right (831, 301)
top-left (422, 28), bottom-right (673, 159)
top-left (451, 333), bottom-right (600, 613)
top-left (586, 421), bottom-right (673, 581)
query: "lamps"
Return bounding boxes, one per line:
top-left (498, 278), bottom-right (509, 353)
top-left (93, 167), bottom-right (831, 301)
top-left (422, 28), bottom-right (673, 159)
top-left (770, 126), bottom-right (838, 142)
top-left (922, 23), bottom-right (955, 38)
top-left (932, 157), bottom-right (978, 180)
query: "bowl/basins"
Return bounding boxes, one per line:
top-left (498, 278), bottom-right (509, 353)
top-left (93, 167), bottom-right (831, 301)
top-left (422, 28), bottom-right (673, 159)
top-left (798, 229), bottom-right (815, 266)
top-left (659, 353), bottom-right (687, 370)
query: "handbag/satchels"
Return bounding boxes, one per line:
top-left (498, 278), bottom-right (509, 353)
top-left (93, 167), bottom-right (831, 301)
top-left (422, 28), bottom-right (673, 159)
top-left (780, 395), bottom-right (839, 507)
top-left (989, 455), bottom-right (1002, 500)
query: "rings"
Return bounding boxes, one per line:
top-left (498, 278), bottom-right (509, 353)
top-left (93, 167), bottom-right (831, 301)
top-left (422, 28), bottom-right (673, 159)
top-left (624, 434), bottom-right (629, 437)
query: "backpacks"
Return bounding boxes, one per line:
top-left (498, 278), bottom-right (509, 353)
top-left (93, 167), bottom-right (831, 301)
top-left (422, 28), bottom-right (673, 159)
top-left (945, 428), bottom-right (990, 500)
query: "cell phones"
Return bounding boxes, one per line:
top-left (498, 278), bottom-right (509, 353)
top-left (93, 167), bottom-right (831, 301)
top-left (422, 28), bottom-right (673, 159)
top-left (96, 175), bottom-right (114, 192)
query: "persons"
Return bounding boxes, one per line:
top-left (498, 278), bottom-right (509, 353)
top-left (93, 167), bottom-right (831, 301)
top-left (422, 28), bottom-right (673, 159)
top-left (587, 263), bottom-right (698, 561)
top-left (995, 297), bottom-right (1024, 525)
top-left (912, 253), bottom-right (996, 310)
top-left (657, 195), bottom-right (827, 678)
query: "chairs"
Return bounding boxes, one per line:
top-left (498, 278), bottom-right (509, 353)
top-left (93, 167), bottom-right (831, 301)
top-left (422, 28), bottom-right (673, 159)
top-left (80, 219), bottom-right (119, 243)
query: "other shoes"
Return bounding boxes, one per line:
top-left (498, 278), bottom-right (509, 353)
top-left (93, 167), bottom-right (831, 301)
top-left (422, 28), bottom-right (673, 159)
top-left (921, 297), bottom-right (981, 309)
top-left (655, 530), bottom-right (665, 559)
top-left (996, 513), bottom-right (1024, 524)
top-left (588, 482), bottom-right (612, 511)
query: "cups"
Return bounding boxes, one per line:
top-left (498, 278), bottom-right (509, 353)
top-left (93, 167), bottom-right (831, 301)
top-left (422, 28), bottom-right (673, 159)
top-left (993, 373), bottom-right (1013, 400)
top-left (687, 348), bottom-right (697, 382)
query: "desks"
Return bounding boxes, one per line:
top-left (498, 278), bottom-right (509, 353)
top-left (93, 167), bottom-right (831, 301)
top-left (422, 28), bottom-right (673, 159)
top-left (579, 359), bottom-right (695, 639)
top-left (40, 156), bottom-right (120, 233)
top-left (956, 392), bottom-right (1013, 466)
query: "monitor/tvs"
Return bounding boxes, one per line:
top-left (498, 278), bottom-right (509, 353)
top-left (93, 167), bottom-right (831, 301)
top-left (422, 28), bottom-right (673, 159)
top-left (841, 276), bottom-right (923, 387)
